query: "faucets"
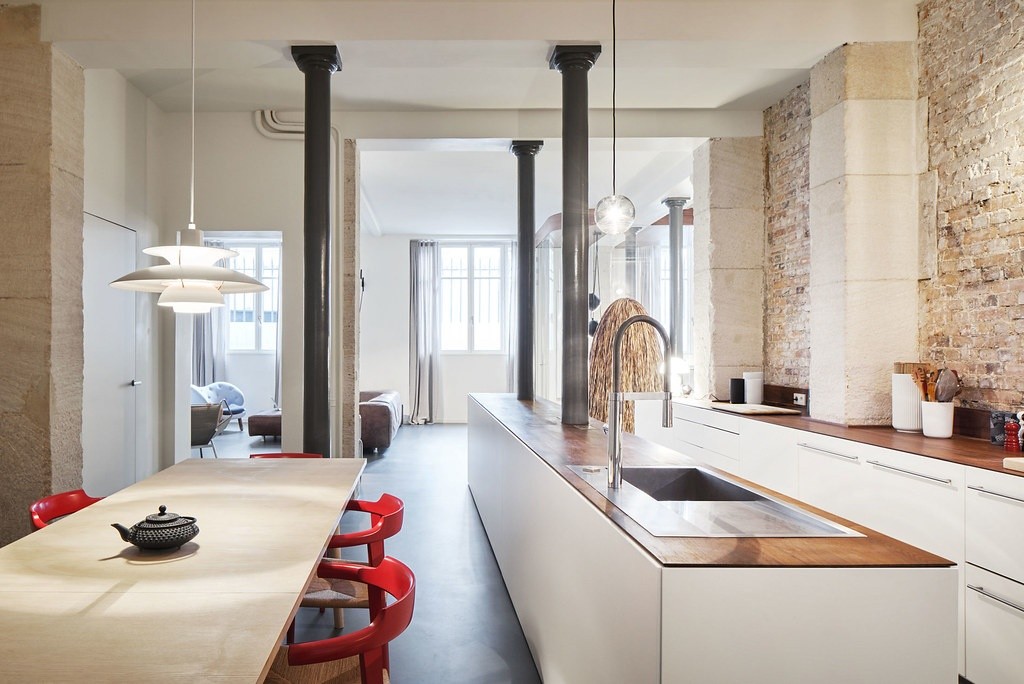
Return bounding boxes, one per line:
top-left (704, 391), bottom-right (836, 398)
top-left (607, 314), bottom-right (673, 489)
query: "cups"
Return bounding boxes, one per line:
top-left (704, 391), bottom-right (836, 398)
top-left (891, 373), bottom-right (922, 433)
top-left (922, 400), bottom-right (954, 439)
top-left (743, 372), bottom-right (764, 404)
top-left (731, 378), bottom-right (745, 404)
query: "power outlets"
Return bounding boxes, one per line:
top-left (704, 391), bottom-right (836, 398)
top-left (794, 393), bottom-right (806, 407)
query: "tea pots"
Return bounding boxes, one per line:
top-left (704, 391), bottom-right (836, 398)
top-left (111, 504), bottom-right (199, 551)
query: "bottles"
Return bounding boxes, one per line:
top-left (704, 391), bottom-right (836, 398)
top-left (1004, 419), bottom-right (1019, 447)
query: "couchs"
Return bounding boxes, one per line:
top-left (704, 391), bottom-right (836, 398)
top-left (359, 390), bottom-right (402, 455)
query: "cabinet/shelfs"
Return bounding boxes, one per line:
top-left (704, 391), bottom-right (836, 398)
top-left (672, 402), bottom-right (740, 477)
top-left (965, 464), bottom-right (1024, 684)
top-left (795, 428), bottom-right (965, 678)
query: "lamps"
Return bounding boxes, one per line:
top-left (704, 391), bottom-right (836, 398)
top-left (593, 0), bottom-right (636, 236)
top-left (106, 0), bottom-right (271, 316)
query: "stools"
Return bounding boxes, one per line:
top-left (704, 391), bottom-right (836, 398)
top-left (263, 557), bottom-right (415, 684)
top-left (286, 492), bottom-right (405, 645)
top-left (29, 488), bottom-right (106, 532)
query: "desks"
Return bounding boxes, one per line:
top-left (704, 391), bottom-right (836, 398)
top-left (248, 407), bottom-right (281, 443)
top-left (0, 456), bottom-right (368, 684)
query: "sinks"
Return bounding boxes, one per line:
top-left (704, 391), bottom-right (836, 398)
top-left (621, 468), bottom-right (773, 502)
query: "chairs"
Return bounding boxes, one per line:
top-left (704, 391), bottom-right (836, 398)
top-left (190, 381), bottom-right (246, 436)
top-left (191, 398), bottom-right (232, 459)
